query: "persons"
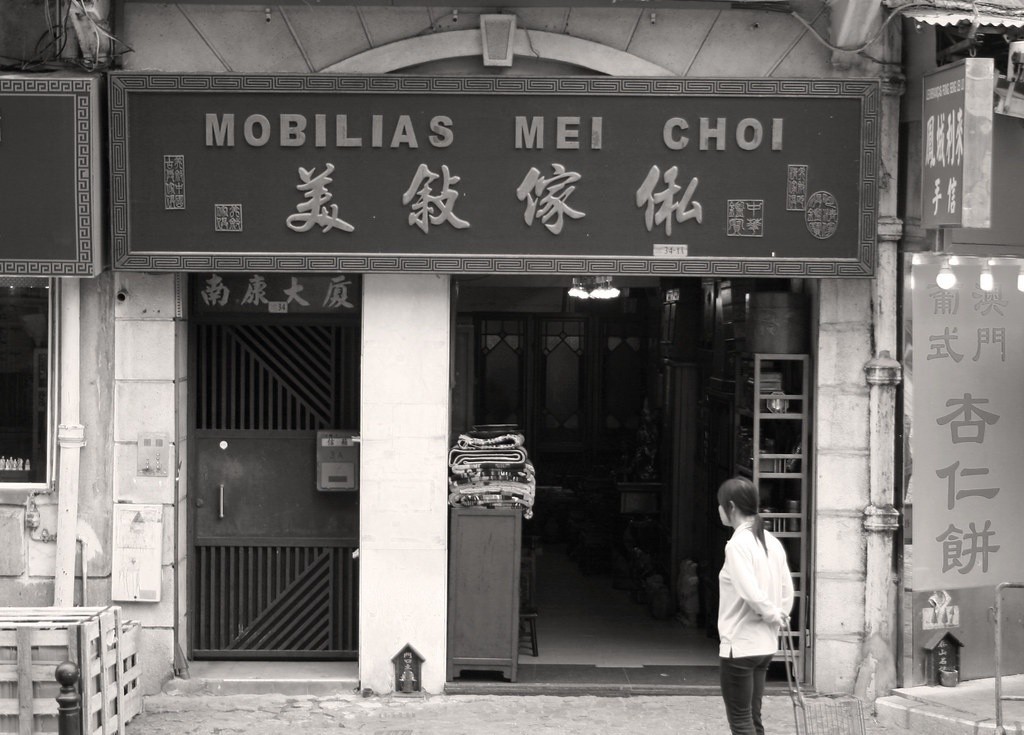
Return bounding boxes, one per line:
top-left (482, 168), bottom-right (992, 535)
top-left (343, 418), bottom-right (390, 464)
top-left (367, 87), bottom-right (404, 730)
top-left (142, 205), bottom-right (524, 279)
top-left (674, 559), bottom-right (699, 626)
top-left (717, 476), bottom-right (795, 735)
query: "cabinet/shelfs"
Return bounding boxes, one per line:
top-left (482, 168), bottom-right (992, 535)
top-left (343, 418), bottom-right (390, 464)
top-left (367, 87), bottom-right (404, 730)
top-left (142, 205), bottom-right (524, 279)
top-left (619, 350), bottom-right (810, 682)
top-left (447, 505), bottom-right (523, 684)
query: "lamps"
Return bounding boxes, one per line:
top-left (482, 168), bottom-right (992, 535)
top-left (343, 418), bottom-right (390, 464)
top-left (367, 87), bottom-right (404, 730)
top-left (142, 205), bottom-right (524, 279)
top-left (568, 276), bottom-right (620, 300)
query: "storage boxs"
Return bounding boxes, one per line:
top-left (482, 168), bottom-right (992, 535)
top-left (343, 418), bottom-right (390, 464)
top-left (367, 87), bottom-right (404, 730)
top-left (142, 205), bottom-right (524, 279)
top-left (658, 278), bottom-right (810, 361)
top-left (0, 604), bottom-right (143, 735)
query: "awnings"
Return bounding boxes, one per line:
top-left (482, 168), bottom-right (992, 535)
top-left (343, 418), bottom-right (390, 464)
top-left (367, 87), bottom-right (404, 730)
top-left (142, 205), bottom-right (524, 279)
top-left (881, 0), bottom-right (1024, 28)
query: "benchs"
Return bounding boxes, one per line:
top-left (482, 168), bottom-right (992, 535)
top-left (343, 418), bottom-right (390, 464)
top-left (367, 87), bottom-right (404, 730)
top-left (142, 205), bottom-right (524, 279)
top-left (519, 534), bottom-right (539, 656)
